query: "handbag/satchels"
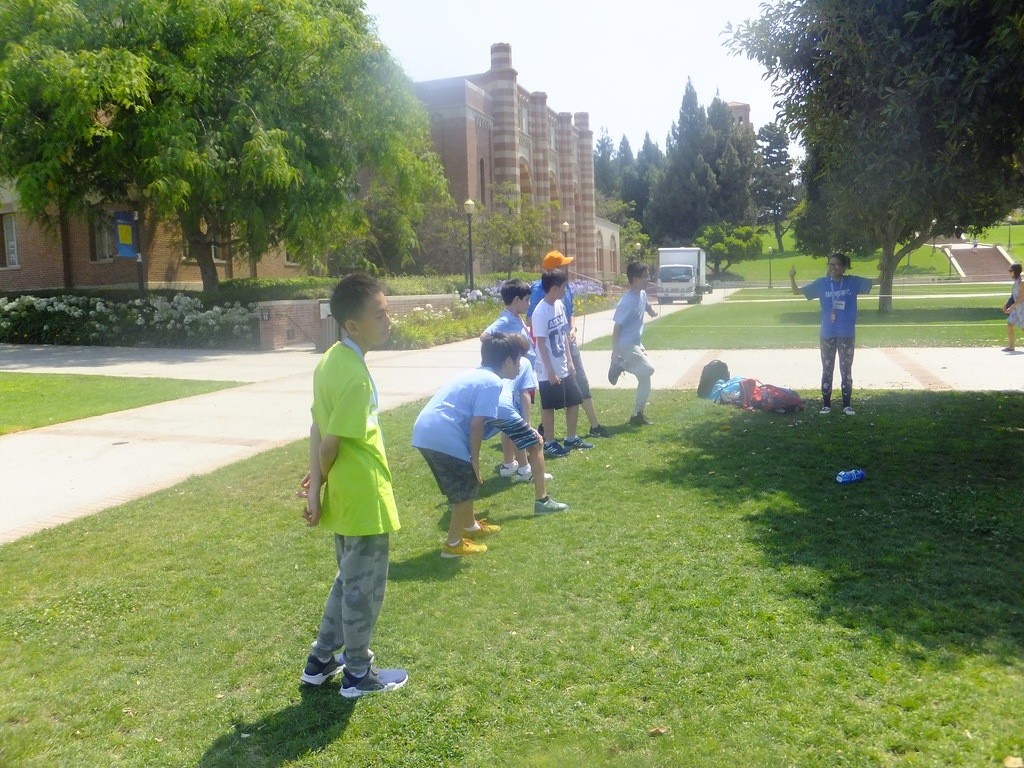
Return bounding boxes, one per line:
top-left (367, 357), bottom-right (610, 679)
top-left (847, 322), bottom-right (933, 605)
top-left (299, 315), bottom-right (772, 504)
top-left (1006, 297), bottom-right (1015, 308)
top-left (746, 384), bottom-right (802, 412)
top-left (711, 376), bottom-right (745, 399)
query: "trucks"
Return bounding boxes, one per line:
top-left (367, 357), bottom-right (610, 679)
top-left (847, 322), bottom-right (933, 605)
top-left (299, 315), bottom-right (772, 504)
top-left (656, 247), bottom-right (706, 304)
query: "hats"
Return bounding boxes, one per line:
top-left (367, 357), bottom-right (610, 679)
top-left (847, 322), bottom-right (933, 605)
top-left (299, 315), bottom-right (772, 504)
top-left (543, 250), bottom-right (574, 269)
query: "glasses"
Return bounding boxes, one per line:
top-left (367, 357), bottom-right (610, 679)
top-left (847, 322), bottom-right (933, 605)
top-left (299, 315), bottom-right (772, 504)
top-left (828, 263), bottom-right (843, 267)
top-left (1009, 269), bottom-right (1014, 273)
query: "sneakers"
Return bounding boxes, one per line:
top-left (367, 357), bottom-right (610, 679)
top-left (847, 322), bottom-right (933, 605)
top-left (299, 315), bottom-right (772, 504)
top-left (500, 462), bottom-right (518, 477)
top-left (515, 467), bottom-right (553, 483)
top-left (460, 517), bottom-right (502, 538)
top-left (441, 536), bottom-right (487, 558)
top-left (537, 424), bottom-right (544, 435)
top-left (628, 412), bottom-right (655, 426)
top-left (535, 496), bottom-right (568, 516)
top-left (301, 649), bottom-right (374, 685)
top-left (589, 425), bottom-right (608, 437)
top-left (609, 358), bottom-right (624, 385)
top-left (339, 665), bottom-right (408, 697)
top-left (544, 442), bottom-right (569, 456)
top-left (563, 437), bottom-right (595, 450)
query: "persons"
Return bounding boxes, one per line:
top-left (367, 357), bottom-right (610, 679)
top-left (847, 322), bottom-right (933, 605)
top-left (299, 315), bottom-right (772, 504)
top-left (1001, 263), bottom-right (1024, 351)
top-left (293, 273), bottom-right (408, 698)
top-left (971, 238), bottom-right (978, 252)
top-left (478, 251), bottom-right (615, 517)
top-left (789, 253), bottom-right (887, 415)
top-left (608, 260), bottom-right (659, 425)
top-left (412, 331), bottom-right (525, 557)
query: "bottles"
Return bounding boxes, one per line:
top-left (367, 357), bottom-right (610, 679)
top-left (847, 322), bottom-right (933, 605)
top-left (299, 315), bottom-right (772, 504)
top-left (836, 469), bottom-right (864, 484)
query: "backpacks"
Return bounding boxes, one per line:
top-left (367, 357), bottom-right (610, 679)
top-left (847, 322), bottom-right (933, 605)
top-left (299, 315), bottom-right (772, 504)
top-left (698, 360), bottom-right (730, 396)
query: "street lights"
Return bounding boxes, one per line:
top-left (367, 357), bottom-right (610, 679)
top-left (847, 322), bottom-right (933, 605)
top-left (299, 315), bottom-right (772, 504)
top-left (768, 247), bottom-right (773, 285)
top-left (949, 243), bottom-right (952, 276)
top-left (636, 242), bottom-right (641, 260)
top-left (1008, 216), bottom-right (1011, 246)
top-left (561, 221), bottom-right (569, 283)
top-left (464, 198), bottom-right (475, 293)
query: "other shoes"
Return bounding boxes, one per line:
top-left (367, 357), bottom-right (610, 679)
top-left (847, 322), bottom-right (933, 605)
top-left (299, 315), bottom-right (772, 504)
top-left (842, 406), bottom-right (855, 415)
top-left (819, 406), bottom-right (831, 414)
top-left (1002, 347), bottom-right (1015, 351)
